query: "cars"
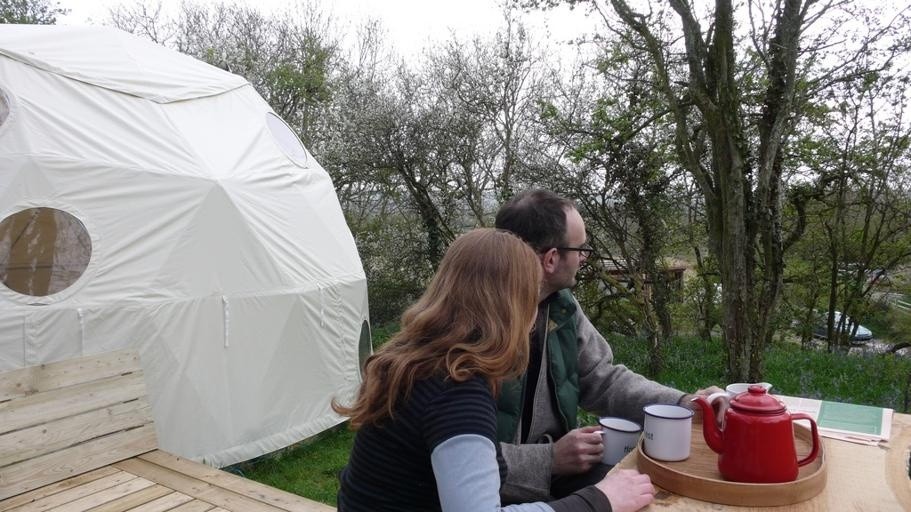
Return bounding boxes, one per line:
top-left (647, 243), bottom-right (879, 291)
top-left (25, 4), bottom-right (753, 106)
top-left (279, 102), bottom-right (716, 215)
top-left (809, 311), bottom-right (873, 345)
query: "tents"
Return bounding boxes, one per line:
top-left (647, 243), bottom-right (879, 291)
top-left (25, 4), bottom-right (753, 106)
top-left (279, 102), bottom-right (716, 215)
top-left (0, 21), bottom-right (376, 470)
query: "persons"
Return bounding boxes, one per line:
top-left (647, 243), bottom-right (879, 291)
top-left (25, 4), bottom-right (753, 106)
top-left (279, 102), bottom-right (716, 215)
top-left (493, 190), bottom-right (729, 506)
top-left (331, 226), bottom-right (656, 511)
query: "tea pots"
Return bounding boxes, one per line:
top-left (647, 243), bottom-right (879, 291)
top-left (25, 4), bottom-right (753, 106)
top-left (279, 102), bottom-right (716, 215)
top-left (690, 382), bottom-right (821, 483)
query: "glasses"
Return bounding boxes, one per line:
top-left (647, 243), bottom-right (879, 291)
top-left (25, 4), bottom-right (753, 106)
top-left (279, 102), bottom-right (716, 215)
top-left (539, 243), bottom-right (595, 258)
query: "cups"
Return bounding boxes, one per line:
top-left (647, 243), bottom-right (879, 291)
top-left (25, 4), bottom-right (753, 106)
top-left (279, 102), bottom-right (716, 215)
top-left (592, 416), bottom-right (641, 466)
top-left (643, 403), bottom-right (695, 461)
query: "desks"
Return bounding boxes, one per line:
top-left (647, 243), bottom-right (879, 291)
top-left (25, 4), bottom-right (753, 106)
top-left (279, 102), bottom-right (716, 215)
top-left (600, 393), bottom-right (911, 512)
